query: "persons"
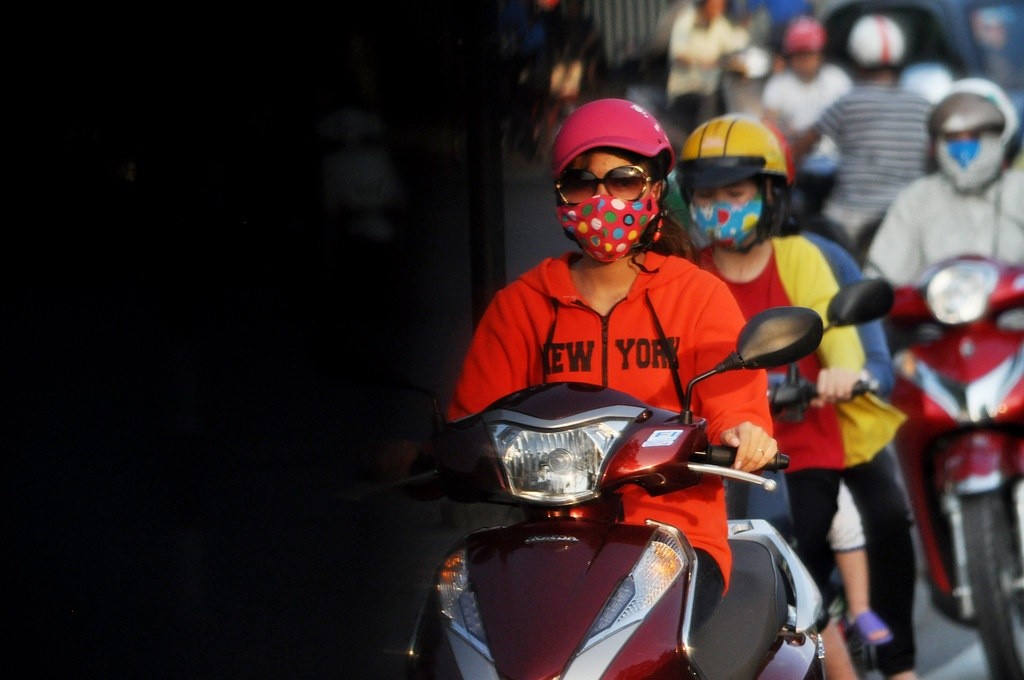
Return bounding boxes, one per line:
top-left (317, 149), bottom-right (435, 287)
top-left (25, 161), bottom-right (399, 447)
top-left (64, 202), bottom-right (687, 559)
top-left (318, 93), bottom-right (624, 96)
top-left (862, 77), bottom-right (1024, 341)
top-left (446, 99), bottom-right (779, 637)
top-left (665, 0), bottom-right (1024, 271)
top-left (674, 117), bottom-right (918, 680)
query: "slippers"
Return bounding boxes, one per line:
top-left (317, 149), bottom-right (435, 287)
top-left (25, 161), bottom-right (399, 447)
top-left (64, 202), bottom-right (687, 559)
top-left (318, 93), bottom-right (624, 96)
top-left (845, 608), bottom-right (894, 647)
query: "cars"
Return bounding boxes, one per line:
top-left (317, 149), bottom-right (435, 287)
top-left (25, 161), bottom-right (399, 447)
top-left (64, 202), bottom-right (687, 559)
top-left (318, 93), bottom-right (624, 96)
top-left (819, 0), bottom-right (1024, 111)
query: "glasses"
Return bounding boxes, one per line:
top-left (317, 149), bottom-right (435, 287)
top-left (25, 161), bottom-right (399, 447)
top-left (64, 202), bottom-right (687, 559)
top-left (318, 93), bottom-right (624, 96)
top-left (556, 166), bottom-right (658, 206)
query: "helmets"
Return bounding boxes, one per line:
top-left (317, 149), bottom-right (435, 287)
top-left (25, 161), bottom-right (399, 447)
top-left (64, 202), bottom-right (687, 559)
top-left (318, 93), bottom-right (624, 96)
top-left (929, 91), bottom-right (1005, 135)
top-left (551, 97), bottom-right (675, 179)
top-left (781, 16), bottom-right (826, 57)
top-left (676, 115), bottom-right (790, 191)
top-left (848, 12), bottom-right (908, 69)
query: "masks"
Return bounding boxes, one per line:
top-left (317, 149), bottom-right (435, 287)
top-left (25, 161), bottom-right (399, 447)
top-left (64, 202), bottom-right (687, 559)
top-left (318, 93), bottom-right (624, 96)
top-left (933, 137), bottom-right (1006, 192)
top-left (553, 189), bottom-right (660, 263)
top-left (687, 189), bottom-right (764, 251)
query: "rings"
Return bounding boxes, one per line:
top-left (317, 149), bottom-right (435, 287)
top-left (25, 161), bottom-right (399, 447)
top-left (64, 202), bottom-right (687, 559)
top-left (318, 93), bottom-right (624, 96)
top-left (757, 447), bottom-right (765, 455)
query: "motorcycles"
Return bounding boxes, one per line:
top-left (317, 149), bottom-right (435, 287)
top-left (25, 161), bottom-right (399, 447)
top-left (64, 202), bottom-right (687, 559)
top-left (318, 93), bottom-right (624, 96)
top-left (372, 307), bottom-right (795, 679)
top-left (499, 49), bottom-right (595, 159)
top-left (717, 275), bottom-right (896, 679)
top-left (887, 248), bottom-right (1024, 680)
